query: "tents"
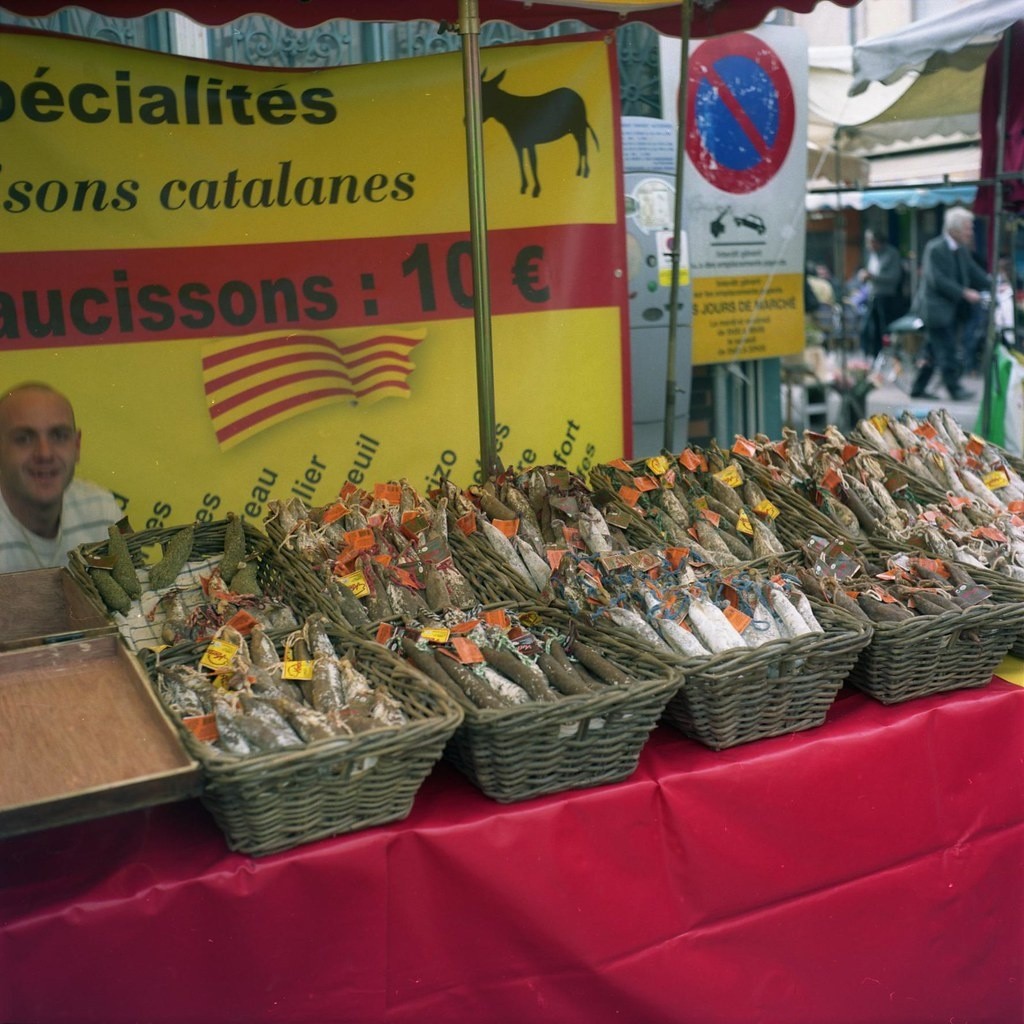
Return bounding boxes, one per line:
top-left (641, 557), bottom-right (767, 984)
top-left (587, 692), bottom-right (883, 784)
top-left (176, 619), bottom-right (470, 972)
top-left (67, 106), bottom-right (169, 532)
top-left (807, 1), bottom-right (1024, 440)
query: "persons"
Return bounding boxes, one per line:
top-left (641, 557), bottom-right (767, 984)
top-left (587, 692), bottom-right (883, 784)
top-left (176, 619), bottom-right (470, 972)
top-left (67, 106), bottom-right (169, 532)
top-left (0, 384), bottom-right (126, 574)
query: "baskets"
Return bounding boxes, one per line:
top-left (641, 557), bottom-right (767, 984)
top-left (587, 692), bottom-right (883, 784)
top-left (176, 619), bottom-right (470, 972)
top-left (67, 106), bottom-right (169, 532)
top-left (61, 432), bottom-right (1022, 859)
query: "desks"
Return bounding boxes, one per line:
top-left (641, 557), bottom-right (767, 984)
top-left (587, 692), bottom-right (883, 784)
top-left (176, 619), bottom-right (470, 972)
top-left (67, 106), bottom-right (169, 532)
top-left (1, 656), bottom-right (1023, 1024)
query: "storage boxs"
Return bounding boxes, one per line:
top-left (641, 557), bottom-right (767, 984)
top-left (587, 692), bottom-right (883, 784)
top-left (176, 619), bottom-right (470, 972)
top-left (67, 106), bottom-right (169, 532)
top-left (870, 531), bottom-right (1024, 655)
top-left (730, 449), bottom-right (953, 545)
top-left (747, 542), bottom-right (1023, 706)
top-left (143, 626), bottom-right (466, 859)
top-left (71, 521), bottom-right (325, 658)
top-left (848, 429), bottom-right (1023, 506)
top-left (354, 600), bottom-right (686, 805)
top-left (262, 493), bottom-right (549, 641)
top-left (437, 467), bottom-right (672, 603)
top-left (547, 565), bottom-right (874, 750)
top-left (588, 451), bottom-right (836, 571)
